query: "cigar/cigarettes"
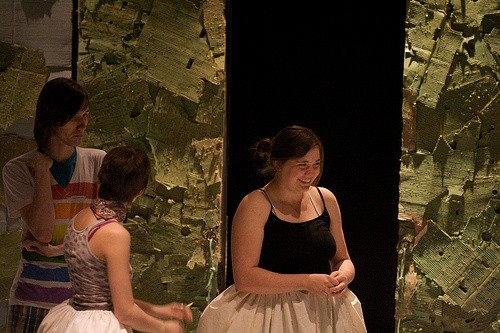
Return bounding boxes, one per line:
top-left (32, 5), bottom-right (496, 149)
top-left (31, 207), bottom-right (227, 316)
top-left (185, 301), bottom-right (194, 309)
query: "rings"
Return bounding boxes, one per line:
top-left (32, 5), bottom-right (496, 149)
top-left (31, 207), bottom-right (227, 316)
top-left (180, 302), bottom-right (184, 311)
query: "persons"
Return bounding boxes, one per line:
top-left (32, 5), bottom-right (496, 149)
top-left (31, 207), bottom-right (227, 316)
top-left (3, 77), bottom-right (107, 333)
top-left (195, 126), bottom-right (368, 333)
top-left (36, 146), bottom-right (194, 333)
